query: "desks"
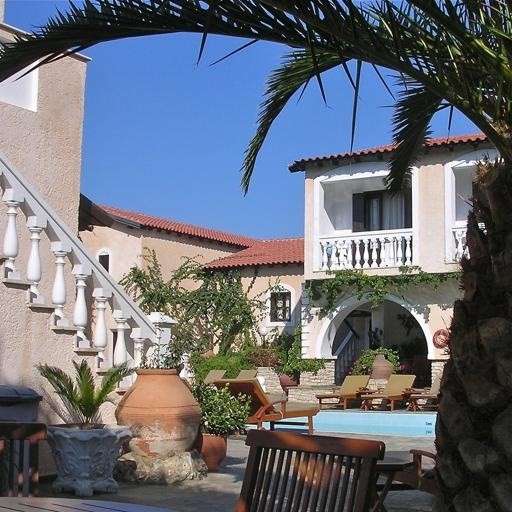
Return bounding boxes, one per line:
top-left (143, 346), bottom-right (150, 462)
top-left (0, 496), bottom-right (184, 512)
top-left (403, 390), bottom-right (422, 412)
top-left (328, 451), bottom-right (416, 512)
top-left (357, 390), bottom-right (377, 411)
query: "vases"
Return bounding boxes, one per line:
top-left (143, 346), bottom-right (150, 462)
top-left (366, 354), bottom-right (394, 386)
top-left (114, 368), bottom-right (203, 456)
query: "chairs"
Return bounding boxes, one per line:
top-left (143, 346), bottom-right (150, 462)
top-left (361, 374), bottom-right (416, 412)
top-left (235, 429), bottom-right (385, 512)
top-left (236, 369), bottom-right (259, 378)
top-left (0, 421), bottom-right (46, 498)
top-left (214, 378), bottom-right (320, 435)
top-left (202, 369), bottom-right (227, 384)
top-left (314, 375), bottom-right (371, 410)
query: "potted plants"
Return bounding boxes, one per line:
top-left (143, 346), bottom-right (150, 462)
top-left (32, 360), bottom-right (137, 497)
top-left (195, 383), bottom-right (252, 472)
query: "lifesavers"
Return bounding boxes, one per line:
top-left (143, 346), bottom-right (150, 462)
top-left (432, 329), bottom-right (449, 349)
